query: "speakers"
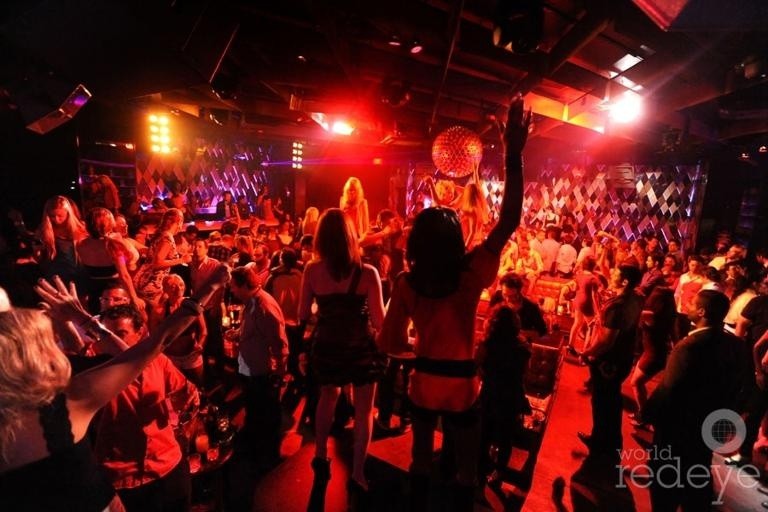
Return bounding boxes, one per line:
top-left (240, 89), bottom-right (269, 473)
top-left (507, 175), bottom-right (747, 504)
top-left (652, 127), bottom-right (692, 165)
top-left (23, 69), bottom-right (91, 134)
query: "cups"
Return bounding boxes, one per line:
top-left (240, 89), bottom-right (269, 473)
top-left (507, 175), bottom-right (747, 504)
top-left (187, 442), bottom-right (220, 474)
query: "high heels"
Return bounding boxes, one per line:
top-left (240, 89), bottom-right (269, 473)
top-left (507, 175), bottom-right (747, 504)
top-left (311, 457), bottom-right (330, 481)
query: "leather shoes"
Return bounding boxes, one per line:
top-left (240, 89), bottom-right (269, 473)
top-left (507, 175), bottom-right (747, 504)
top-left (627, 413), bottom-right (634, 419)
top-left (756, 487), bottom-right (767, 495)
top-left (577, 431), bottom-right (591, 448)
top-left (724, 453), bottom-right (752, 465)
top-left (630, 420), bottom-right (643, 427)
top-left (761, 502), bottom-right (767, 508)
top-left (347, 478), bottom-right (379, 496)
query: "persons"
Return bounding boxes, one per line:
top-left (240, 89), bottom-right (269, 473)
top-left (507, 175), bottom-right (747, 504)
top-left (2, 97), bottom-right (767, 510)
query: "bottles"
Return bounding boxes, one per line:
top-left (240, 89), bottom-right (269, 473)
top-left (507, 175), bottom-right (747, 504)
top-left (193, 420), bottom-right (209, 456)
